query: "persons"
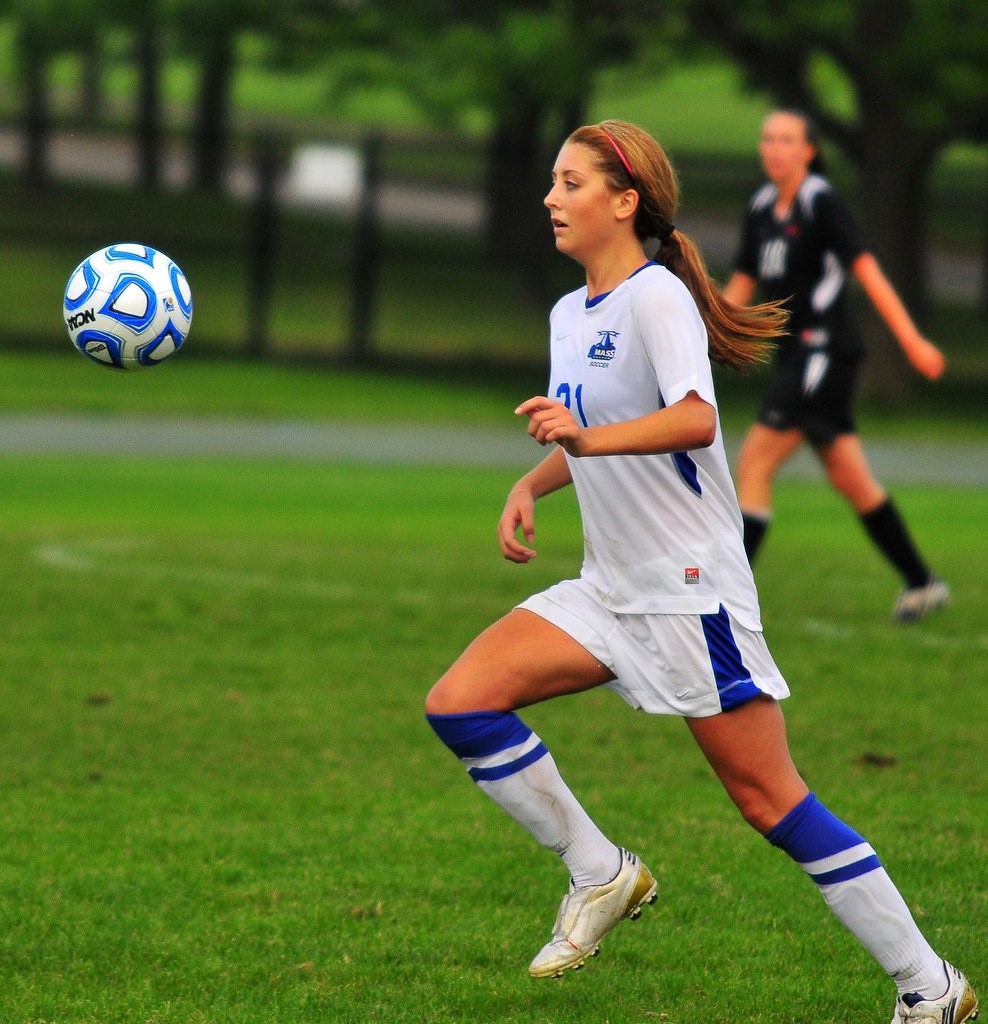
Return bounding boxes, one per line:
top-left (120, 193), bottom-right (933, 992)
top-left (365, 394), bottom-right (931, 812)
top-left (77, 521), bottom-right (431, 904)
top-left (723, 106), bottom-right (950, 621)
top-left (424, 119), bottom-right (979, 1024)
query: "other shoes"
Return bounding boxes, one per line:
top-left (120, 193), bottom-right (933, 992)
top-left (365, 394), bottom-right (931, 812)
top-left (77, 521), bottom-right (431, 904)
top-left (897, 584), bottom-right (949, 618)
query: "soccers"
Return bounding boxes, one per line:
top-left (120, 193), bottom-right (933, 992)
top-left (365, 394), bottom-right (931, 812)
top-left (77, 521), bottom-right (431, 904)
top-left (62, 243), bottom-right (194, 373)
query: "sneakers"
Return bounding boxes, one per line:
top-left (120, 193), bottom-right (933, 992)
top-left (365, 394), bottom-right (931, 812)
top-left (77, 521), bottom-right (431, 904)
top-left (889, 959), bottom-right (979, 1024)
top-left (529, 846), bottom-right (658, 979)
